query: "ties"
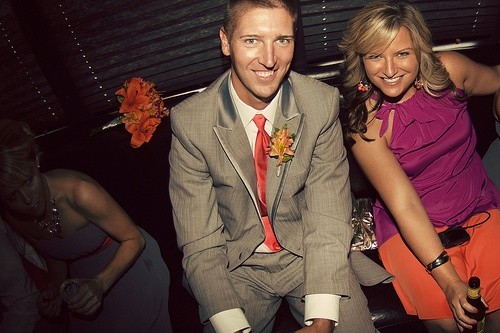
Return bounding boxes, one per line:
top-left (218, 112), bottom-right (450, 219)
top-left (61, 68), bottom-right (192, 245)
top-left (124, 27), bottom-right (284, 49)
top-left (252, 114), bottom-right (283, 252)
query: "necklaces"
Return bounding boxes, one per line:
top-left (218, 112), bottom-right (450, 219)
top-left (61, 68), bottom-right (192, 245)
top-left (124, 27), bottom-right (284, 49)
top-left (34, 195), bottom-right (63, 238)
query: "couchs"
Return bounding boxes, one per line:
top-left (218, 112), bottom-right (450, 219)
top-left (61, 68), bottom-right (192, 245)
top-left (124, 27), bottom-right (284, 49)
top-left (64, 48), bottom-right (500, 333)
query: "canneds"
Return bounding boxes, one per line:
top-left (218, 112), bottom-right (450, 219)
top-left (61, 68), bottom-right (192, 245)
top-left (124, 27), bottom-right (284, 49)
top-left (59, 281), bottom-right (80, 303)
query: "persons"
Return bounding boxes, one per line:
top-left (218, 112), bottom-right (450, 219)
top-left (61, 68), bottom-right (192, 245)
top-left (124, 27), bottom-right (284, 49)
top-left (168, 0), bottom-right (395, 333)
top-left (1, 119), bottom-right (173, 333)
top-left (332, 0), bottom-right (500, 333)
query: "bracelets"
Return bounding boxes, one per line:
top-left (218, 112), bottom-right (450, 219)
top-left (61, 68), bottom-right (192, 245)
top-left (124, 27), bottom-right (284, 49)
top-left (427, 251), bottom-right (451, 271)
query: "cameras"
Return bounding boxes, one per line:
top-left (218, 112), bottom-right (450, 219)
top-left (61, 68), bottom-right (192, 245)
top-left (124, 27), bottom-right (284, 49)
top-left (437, 226), bottom-right (471, 249)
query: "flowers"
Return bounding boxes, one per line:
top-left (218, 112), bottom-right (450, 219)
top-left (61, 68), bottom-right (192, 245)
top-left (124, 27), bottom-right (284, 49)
top-left (116, 76), bottom-right (168, 147)
top-left (268, 123), bottom-right (296, 176)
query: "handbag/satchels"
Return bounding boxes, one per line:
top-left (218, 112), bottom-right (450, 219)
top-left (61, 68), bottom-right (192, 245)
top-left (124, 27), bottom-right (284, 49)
top-left (349, 197), bottom-right (378, 252)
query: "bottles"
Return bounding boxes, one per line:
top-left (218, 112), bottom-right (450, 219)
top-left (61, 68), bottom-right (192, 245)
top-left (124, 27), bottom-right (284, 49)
top-left (459, 276), bottom-right (486, 333)
